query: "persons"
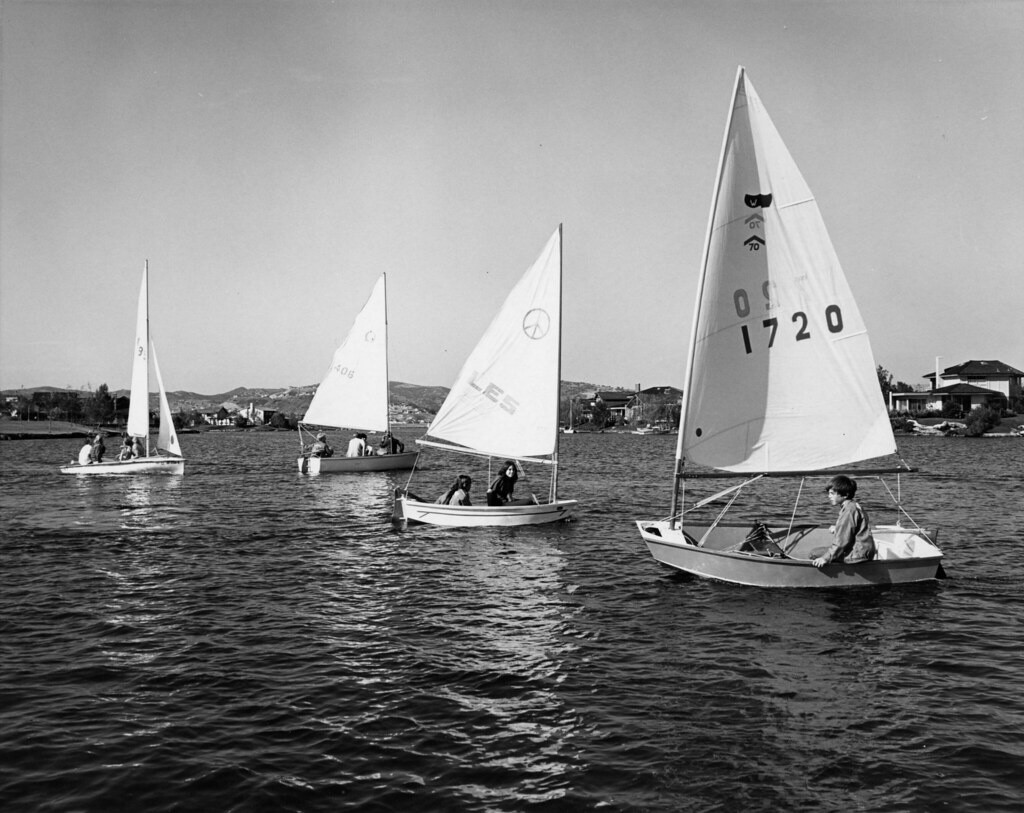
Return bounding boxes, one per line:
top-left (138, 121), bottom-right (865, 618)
top-left (78, 432), bottom-right (146, 466)
top-left (486, 460), bottom-right (534, 506)
top-left (312, 433), bottom-right (334, 459)
top-left (812, 476), bottom-right (877, 568)
top-left (434, 475), bottom-right (472, 507)
top-left (346, 431), bottom-right (405, 457)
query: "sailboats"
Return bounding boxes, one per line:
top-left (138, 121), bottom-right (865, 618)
top-left (60, 258), bottom-right (188, 477)
top-left (296, 271), bottom-right (420, 474)
top-left (634, 65), bottom-right (945, 589)
top-left (392, 222), bottom-right (579, 530)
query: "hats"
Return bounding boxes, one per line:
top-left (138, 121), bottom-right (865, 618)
top-left (317, 433), bottom-right (326, 440)
